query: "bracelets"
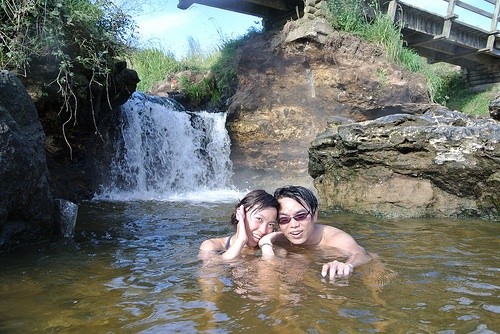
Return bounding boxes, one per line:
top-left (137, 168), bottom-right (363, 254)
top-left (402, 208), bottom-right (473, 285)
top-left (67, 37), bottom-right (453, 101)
top-left (259, 243), bottom-right (274, 248)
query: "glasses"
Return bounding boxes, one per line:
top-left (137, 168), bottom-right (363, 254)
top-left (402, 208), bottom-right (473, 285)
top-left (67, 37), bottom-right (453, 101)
top-left (278, 211), bottom-right (312, 226)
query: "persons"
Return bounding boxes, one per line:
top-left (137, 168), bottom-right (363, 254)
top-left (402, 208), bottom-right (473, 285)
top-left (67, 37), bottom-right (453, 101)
top-left (272, 185), bottom-right (374, 278)
top-left (198, 190), bottom-right (289, 261)
